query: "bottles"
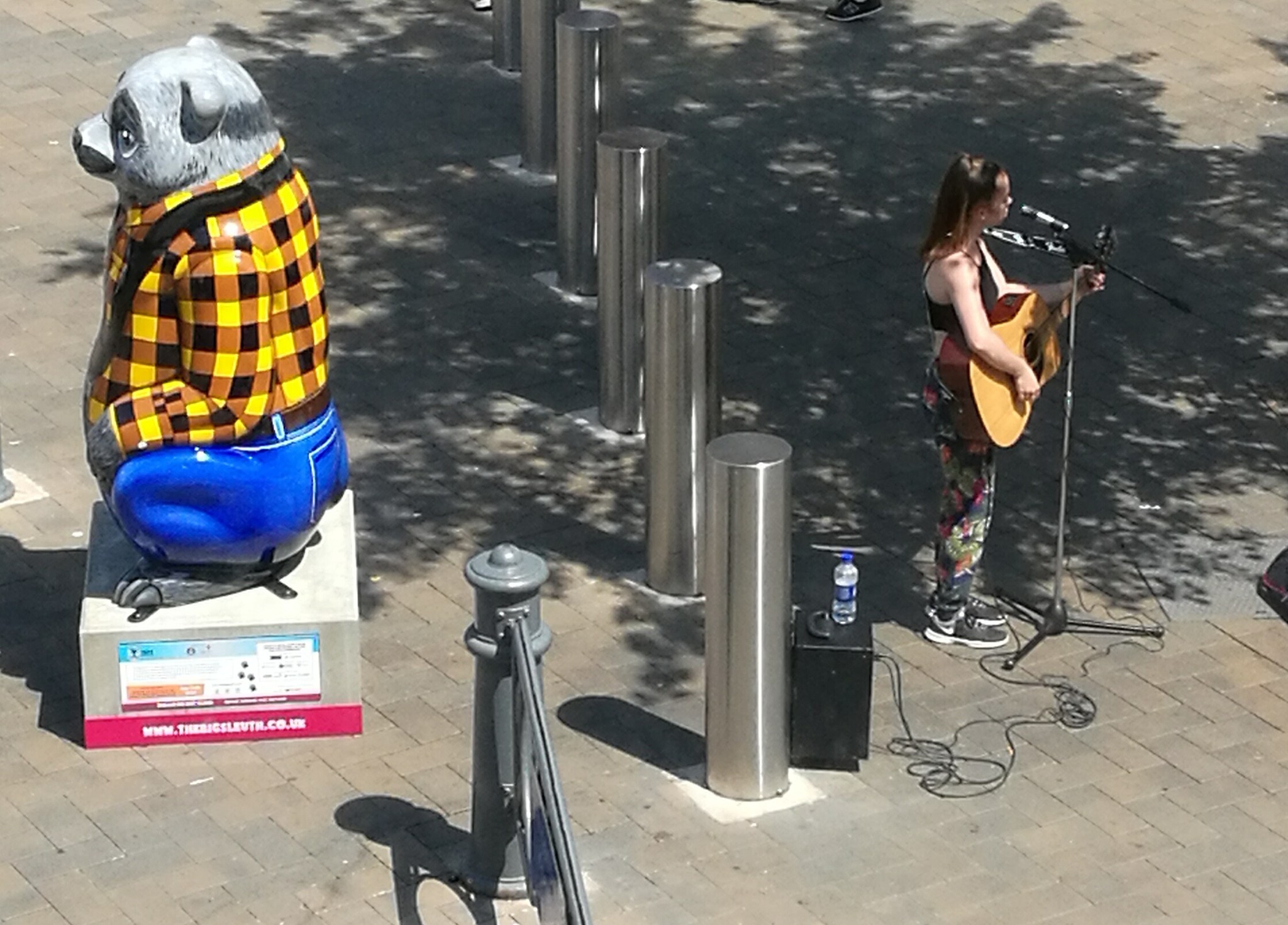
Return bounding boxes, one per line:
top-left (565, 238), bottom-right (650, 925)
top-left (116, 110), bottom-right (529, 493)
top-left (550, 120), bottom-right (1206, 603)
top-left (830, 554), bottom-right (859, 626)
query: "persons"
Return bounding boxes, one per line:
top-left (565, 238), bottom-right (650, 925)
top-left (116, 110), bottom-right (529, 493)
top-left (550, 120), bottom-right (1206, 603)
top-left (824, 0), bottom-right (885, 20)
top-left (920, 155), bottom-right (1104, 647)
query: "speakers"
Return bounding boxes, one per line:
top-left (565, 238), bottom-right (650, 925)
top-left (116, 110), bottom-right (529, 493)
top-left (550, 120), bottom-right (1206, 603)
top-left (786, 607), bottom-right (873, 772)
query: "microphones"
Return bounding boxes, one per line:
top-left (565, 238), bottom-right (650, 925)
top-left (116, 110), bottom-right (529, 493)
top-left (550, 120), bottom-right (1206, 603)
top-left (1018, 204), bottom-right (1070, 230)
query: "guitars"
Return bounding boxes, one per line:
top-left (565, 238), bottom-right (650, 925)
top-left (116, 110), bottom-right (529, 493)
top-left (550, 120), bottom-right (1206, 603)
top-left (938, 222), bottom-right (1121, 449)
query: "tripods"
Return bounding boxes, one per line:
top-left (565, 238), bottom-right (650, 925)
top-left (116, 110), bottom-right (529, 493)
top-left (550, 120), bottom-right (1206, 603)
top-left (995, 230), bottom-right (1195, 671)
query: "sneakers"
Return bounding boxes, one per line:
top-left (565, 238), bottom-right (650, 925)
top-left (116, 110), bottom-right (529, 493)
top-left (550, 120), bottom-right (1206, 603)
top-left (824, 0), bottom-right (884, 23)
top-left (922, 606), bottom-right (1009, 649)
top-left (923, 589), bottom-right (1009, 626)
